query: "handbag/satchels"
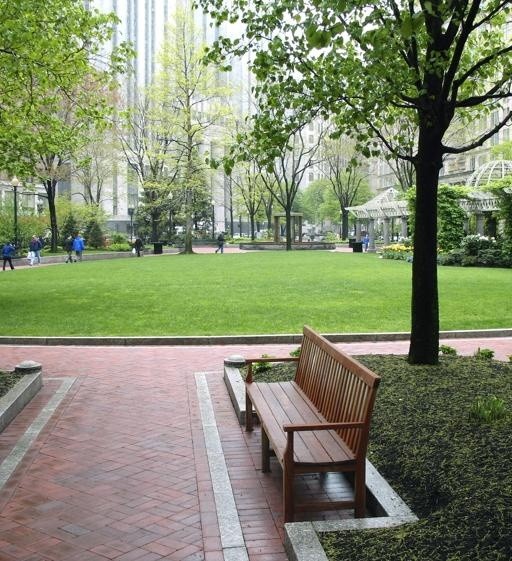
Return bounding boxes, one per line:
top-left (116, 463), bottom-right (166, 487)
top-left (27, 251), bottom-right (31, 258)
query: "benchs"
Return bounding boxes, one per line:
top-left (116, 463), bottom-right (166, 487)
top-left (245, 324), bottom-right (382, 522)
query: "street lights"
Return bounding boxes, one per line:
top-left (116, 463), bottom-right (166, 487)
top-left (11, 177), bottom-right (19, 245)
top-left (339, 209), bottom-right (343, 238)
top-left (127, 202), bottom-right (136, 242)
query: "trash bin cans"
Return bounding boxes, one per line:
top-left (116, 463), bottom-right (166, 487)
top-left (349, 239), bottom-right (356, 247)
top-left (353, 242), bottom-right (362, 252)
top-left (154, 243), bottom-right (162, 253)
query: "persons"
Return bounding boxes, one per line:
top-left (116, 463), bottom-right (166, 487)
top-left (63, 234), bottom-right (73, 263)
top-left (363, 235), bottom-right (369, 251)
top-left (2, 242), bottom-right (15, 270)
top-left (71, 233), bottom-right (86, 262)
top-left (134, 239), bottom-right (144, 257)
top-left (215, 231), bottom-right (226, 254)
top-left (29, 235), bottom-right (42, 266)
